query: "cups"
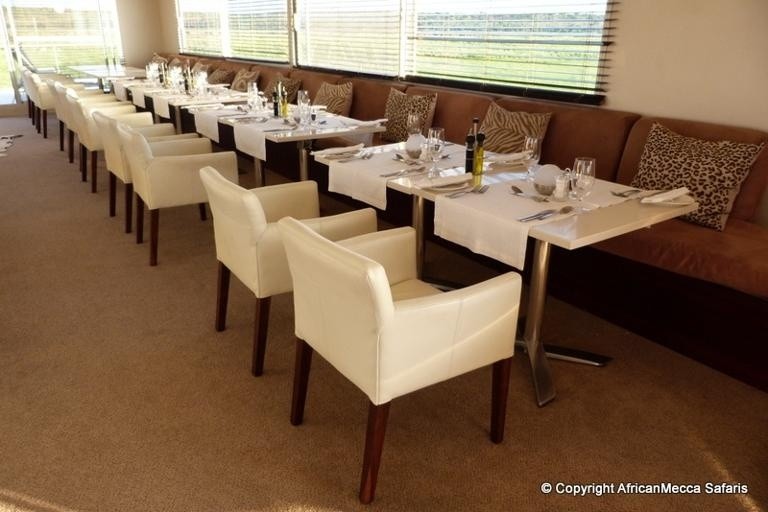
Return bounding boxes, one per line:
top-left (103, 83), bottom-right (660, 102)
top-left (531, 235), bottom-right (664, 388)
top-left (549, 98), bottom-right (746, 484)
top-left (296, 90), bottom-right (312, 126)
top-left (144, 58), bottom-right (209, 98)
top-left (246, 80), bottom-right (258, 108)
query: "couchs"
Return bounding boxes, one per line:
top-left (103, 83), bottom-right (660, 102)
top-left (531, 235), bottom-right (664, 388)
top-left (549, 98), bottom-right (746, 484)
top-left (147, 49), bottom-right (767, 390)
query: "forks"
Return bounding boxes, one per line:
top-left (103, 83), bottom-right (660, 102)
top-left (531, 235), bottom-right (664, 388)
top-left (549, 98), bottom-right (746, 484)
top-left (446, 183), bottom-right (489, 199)
top-left (612, 188), bottom-right (641, 198)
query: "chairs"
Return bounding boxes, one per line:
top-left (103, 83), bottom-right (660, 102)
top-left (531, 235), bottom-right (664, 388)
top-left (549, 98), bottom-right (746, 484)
top-left (277, 214), bottom-right (523, 506)
top-left (22, 72), bottom-right (379, 376)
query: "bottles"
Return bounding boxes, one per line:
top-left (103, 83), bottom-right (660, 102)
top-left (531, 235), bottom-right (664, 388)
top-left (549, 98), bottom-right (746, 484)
top-left (272, 85), bottom-right (289, 117)
top-left (464, 128), bottom-right (486, 177)
top-left (552, 174), bottom-right (570, 202)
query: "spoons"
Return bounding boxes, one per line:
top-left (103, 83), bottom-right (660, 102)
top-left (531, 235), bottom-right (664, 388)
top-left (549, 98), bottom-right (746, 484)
top-left (518, 209), bottom-right (553, 222)
top-left (508, 184), bottom-right (549, 203)
top-left (539, 205), bottom-right (576, 221)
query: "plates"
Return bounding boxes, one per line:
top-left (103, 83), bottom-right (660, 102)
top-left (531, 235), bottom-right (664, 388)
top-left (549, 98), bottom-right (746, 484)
top-left (488, 151), bottom-right (531, 166)
top-left (227, 116), bottom-right (267, 126)
top-left (637, 190), bottom-right (695, 207)
top-left (429, 180), bottom-right (468, 193)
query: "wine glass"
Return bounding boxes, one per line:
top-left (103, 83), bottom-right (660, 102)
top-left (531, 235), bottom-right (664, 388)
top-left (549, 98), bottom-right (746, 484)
top-left (571, 154), bottom-right (597, 212)
top-left (426, 126), bottom-right (446, 172)
top-left (406, 111), bottom-right (423, 136)
top-left (519, 132), bottom-right (542, 183)
